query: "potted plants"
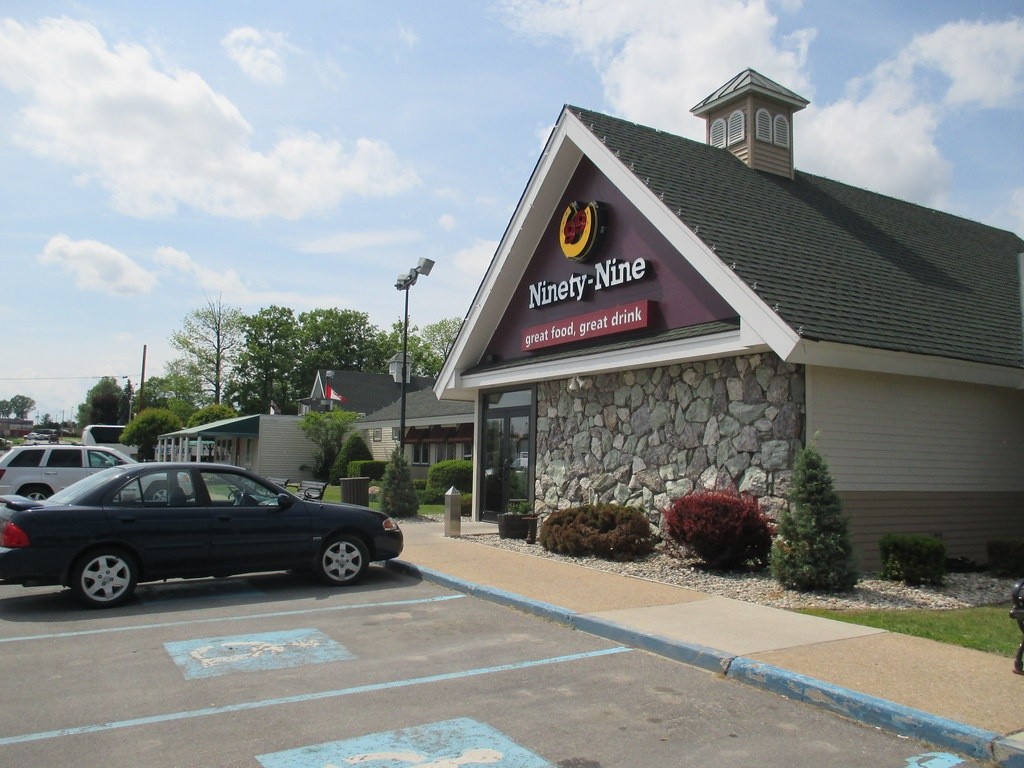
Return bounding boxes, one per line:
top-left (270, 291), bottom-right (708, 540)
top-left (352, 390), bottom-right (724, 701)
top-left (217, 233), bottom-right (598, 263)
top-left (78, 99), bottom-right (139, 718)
top-left (497, 500), bottom-right (537, 541)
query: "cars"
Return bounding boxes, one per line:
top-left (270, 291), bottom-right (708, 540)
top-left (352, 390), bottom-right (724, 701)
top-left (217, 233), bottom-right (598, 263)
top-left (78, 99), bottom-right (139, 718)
top-left (24, 428), bottom-right (60, 444)
top-left (1, 462), bottom-right (406, 610)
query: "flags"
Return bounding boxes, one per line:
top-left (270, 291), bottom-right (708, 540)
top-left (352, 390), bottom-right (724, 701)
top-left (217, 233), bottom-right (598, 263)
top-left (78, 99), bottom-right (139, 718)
top-left (325, 383), bottom-right (346, 402)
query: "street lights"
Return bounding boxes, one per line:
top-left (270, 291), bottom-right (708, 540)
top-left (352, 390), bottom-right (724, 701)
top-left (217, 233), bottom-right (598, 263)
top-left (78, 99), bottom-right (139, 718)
top-left (396, 256), bottom-right (435, 458)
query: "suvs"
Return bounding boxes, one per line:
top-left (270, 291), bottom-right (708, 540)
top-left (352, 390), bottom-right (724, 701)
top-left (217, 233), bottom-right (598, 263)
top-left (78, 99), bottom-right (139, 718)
top-left (82, 424), bottom-right (178, 462)
top-left (0, 442), bottom-right (194, 512)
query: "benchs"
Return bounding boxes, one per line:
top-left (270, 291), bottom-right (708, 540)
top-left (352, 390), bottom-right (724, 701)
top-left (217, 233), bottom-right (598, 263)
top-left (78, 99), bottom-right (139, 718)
top-left (263, 476), bottom-right (290, 497)
top-left (285, 479), bottom-right (328, 501)
